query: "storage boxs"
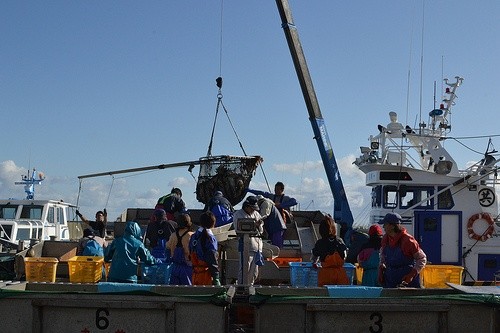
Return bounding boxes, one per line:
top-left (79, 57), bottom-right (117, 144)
top-left (318, 262), bottom-right (356, 286)
top-left (266, 257), bottom-right (302, 267)
top-left (24, 257), bottom-right (59, 283)
top-left (68, 256), bottom-right (104, 283)
top-left (105, 261), bottom-right (112, 281)
top-left (418, 265), bottom-right (464, 289)
top-left (288, 261), bottom-right (321, 288)
top-left (354, 263), bottom-right (365, 284)
top-left (327, 285), bottom-right (383, 298)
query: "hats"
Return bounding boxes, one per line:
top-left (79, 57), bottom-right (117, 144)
top-left (152, 208), bottom-right (166, 215)
top-left (378, 212), bottom-right (403, 224)
top-left (368, 224), bottom-right (383, 236)
top-left (245, 195), bottom-right (260, 210)
top-left (84, 228), bottom-right (95, 234)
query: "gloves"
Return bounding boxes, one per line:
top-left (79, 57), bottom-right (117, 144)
top-left (103, 208), bottom-right (107, 216)
top-left (76, 210), bottom-right (82, 218)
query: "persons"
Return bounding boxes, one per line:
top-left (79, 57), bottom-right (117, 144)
top-left (74, 179), bottom-right (429, 292)
